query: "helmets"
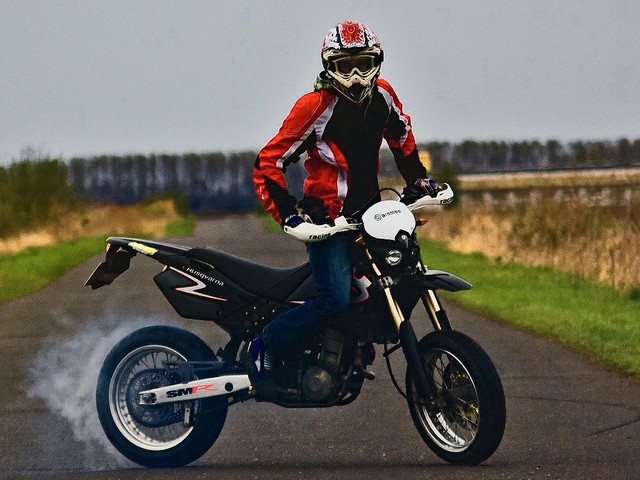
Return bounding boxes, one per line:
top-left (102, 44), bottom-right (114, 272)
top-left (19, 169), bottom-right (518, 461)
top-left (322, 21), bottom-right (383, 104)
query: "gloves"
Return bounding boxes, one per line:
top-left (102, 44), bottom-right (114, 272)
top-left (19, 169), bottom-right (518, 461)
top-left (283, 212), bottom-right (316, 227)
top-left (410, 177), bottom-right (443, 199)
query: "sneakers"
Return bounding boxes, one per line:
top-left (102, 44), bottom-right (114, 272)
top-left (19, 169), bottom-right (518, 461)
top-left (240, 337), bottom-right (279, 401)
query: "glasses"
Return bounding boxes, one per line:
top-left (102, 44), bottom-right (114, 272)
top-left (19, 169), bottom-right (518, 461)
top-left (322, 50), bottom-right (384, 79)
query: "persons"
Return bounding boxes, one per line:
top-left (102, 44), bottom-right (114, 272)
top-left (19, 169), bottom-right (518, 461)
top-left (239, 21), bottom-right (442, 393)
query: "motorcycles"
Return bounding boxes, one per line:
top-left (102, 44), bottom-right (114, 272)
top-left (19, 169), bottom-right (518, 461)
top-left (82, 181), bottom-right (507, 469)
